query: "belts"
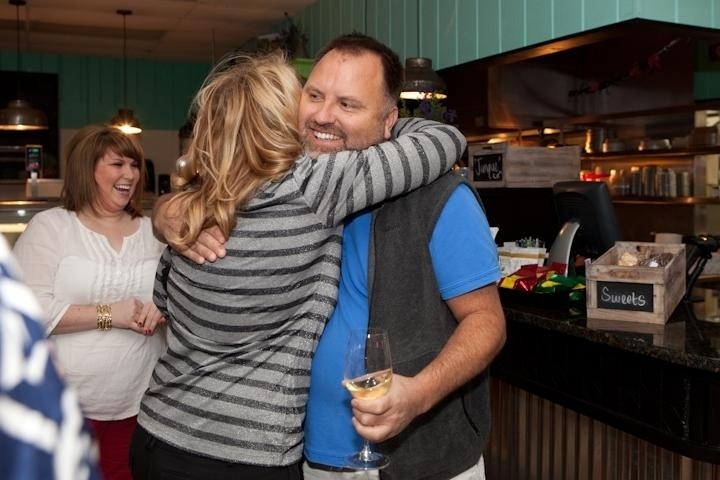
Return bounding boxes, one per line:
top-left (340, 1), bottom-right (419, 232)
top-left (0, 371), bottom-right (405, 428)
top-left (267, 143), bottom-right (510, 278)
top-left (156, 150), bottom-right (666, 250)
top-left (306, 459), bottom-right (356, 473)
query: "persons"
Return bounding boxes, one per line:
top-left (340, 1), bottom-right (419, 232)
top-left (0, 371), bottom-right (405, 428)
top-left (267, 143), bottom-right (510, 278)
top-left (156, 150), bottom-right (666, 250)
top-left (8, 120), bottom-right (172, 480)
top-left (146, 22), bottom-right (510, 480)
top-left (0, 218), bottom-right (102, 480)
top-left (123, 49), bottom-right (470, 480)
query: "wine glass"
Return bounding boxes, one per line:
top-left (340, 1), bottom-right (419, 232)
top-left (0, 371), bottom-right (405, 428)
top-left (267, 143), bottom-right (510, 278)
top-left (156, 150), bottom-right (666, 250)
top-left (342, 326), bottom-right (395, 472)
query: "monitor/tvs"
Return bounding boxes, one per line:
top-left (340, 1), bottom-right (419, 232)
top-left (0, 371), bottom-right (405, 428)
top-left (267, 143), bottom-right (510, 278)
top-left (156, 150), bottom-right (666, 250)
top-left (548, 180), bottom-right (619, 277)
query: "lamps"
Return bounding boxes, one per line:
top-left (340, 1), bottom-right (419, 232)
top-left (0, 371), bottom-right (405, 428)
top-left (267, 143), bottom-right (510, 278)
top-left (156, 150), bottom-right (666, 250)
top-left (108, 10), bottom-right (143, 134)
top-left (0, 0), bottom-right (48, 130)
top-left (399, 0), bottom-right (448, 100)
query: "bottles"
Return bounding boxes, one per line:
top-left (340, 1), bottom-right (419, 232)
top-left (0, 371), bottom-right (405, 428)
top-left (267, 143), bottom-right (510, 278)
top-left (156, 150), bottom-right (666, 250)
top-left (608, 163), bottom-right (694, 202)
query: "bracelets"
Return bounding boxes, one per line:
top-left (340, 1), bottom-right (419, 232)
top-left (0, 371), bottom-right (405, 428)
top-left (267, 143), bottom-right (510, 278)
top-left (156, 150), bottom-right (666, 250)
top-left (96, 301), bottom-right (114, 333)
